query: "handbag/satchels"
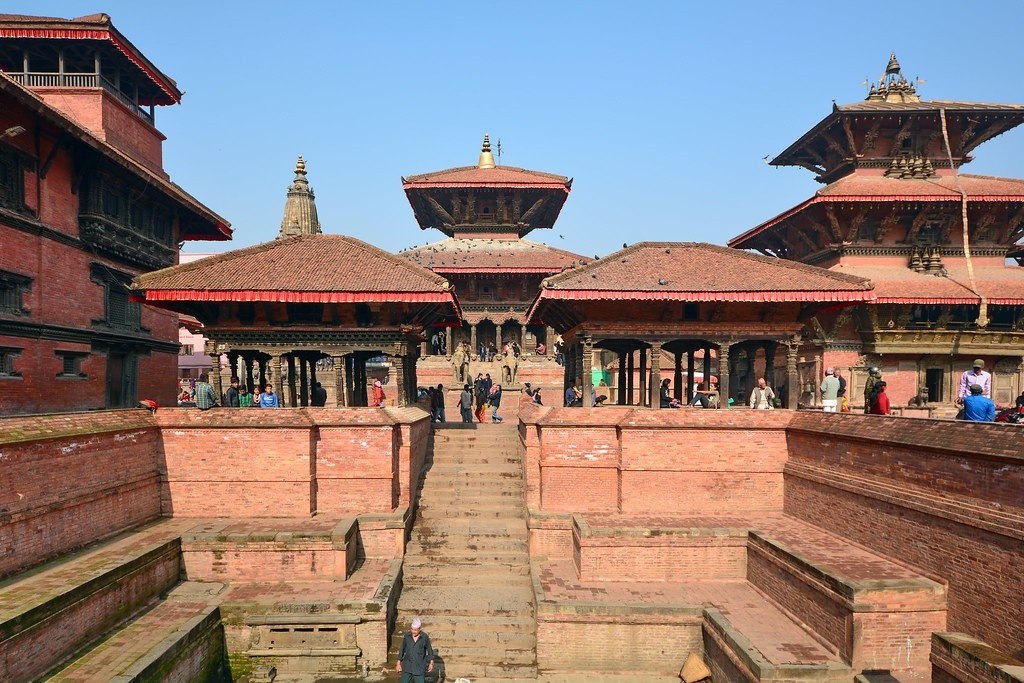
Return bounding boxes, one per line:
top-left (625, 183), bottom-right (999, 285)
top-left (669, 401), bottom-right (681, 408)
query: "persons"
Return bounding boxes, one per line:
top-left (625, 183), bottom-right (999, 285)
top-left (237, 384), bottom-right (253, 407)
top-left (252, 385), bottom-right (261, 407)
top-left (686, 382), bottom-right (720, 409)
top-left (225, 376), bottom-right (240, 407)
top-left (316, 357), bottom-right (333, 372)
top-left (957, 383), bottom-right (995, 422)
top-left (999, 389), bottom-right (1024, 424)
top-left (749, 378), bottom-right (775, 409)
top-left (396, 617), bottom-right (434, 683)
top-left (177, 377), bottom-right (196, 406)
top-left (371, 377), bottom-right (379, 403)
top-left (832, 367), bottom-right (846, 412)
top-left (195, 373), bottom-right (220, 409)
top-left (418, 332), bottom-right (608, 426)
top-left (259, 383), bottom-right (278, 408)
top-left (375, 381), bottom-right (382, 404)
top-left (660, 378), bottom-right (678, 408)
top-left (660, 380), bottom-right (662, 388)
top-left (869, 380), bottom-right (890, 415)
top-left (820, 367), bottom-right (840, 412)
top-left (310, 382), bottom-right (327, 406)
top-left (957, 359), bottom-right (992, 399)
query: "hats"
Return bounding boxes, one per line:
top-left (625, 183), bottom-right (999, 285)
top-left (826, 368), bottom-right (833, 375)
top-left (973, 359), bottom-right (984, 369)
top-left (374, 381), bottom-right (381, 387)
top-left (971, 384), bottom-right (983, 393)
top-left (411, 618), bottom-right (421, 629)
